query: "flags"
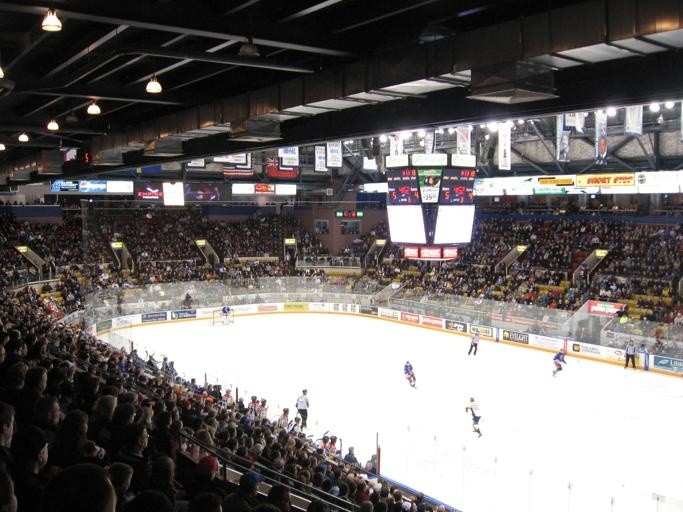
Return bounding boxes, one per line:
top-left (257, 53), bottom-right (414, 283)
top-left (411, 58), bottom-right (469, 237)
top-left (265, 156), bottom-right (297, 179)
top-left (129, 165), bottom-right (161, 175)
top-left (221, 155), bottom-right (255, 178)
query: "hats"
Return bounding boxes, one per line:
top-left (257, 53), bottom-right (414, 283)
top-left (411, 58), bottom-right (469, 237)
top-left (175, 377), bottom-right (447, 511)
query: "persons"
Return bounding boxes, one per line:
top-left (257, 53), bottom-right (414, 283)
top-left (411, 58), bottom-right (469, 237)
top-left (0, 197), bottom-right (311, 428)
top-left (596, 136), bottom-right (608, 166)
top-left (551, 347), bottom-right (567, 378)
top-left (558, 134), bottom-right (571, 162)
top-left (402, 360), bottom-right (416, 389)
top-left (0, 417), bottom-right (451, 511)
top-left (465, 397), bottom-right (482, 438)
top-left (622, 340), bottom-right (636, 370)
top-left (304, 195), bottom-right (683, 354)
top-left (185, 182), bottom-right (220, 202)
top-left (468, 333), bottom-right (480, 355)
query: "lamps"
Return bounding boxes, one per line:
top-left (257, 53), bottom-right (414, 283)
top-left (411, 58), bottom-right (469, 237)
top-left (0, 9), bottom-right (164, 151)
